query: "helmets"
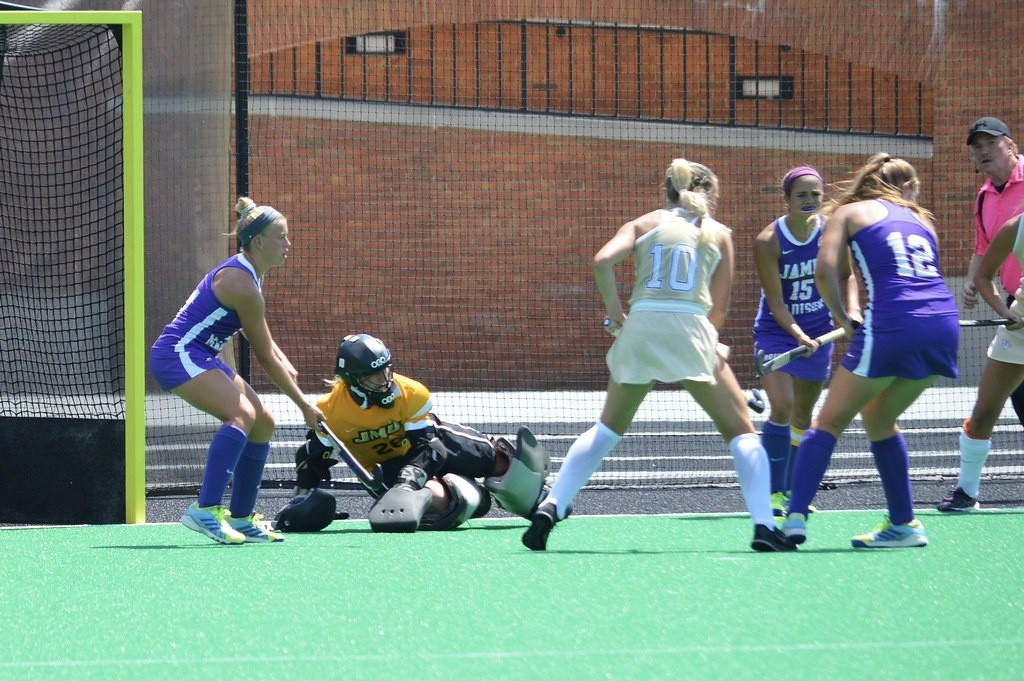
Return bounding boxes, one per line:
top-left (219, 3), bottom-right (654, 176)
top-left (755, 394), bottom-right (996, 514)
top-left (334, 332), bottom-right (395, 409)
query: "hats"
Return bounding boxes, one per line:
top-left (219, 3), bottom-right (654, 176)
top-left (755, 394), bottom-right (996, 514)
top-left (966, 116), bottom-right (1011, 146)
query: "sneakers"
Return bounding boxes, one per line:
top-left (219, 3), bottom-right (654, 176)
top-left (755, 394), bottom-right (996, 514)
top-left (936, 487), bottom-right (980, 513)
top-left (751, 523), bottom-right (797, 552)
top-left (521, 501), bottom-right (557, 551)
top-left (769, 492), bottom-right (816, 517)
top-left (178, 501), bottom-right (246, 543)
top-left (777, 514), bottom-right (807, 544)
top-left (224, 510), bottom-right (285, 543)
top-left (851, 514), bottom-right (928, 548)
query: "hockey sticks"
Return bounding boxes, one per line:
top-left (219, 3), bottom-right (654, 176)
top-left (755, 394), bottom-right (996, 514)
top-left (755, 311), bottom-right (857, 377)
top-left (961, 318), bottom-right (1011, 327)
top-left (282, 376), bottom-right (386, 491)
top-left (603, 317), bottom-right (766, 414)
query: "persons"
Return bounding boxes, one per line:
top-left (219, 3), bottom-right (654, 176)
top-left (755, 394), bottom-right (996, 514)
top-left (963, 116), bottom-right (1024, 425)
top-left (751, 167), bottom-right (864, 522)
top-left (522, 159), bottom-right (798, 552)
top-left (151, 197), bottom-right (327, 544)
top-left (783, 153), bottom-right (958, 548)
top-left (936, 213), bottom-right (1024, 511)
top-left (273, 333), bottom-right (572, 533)
